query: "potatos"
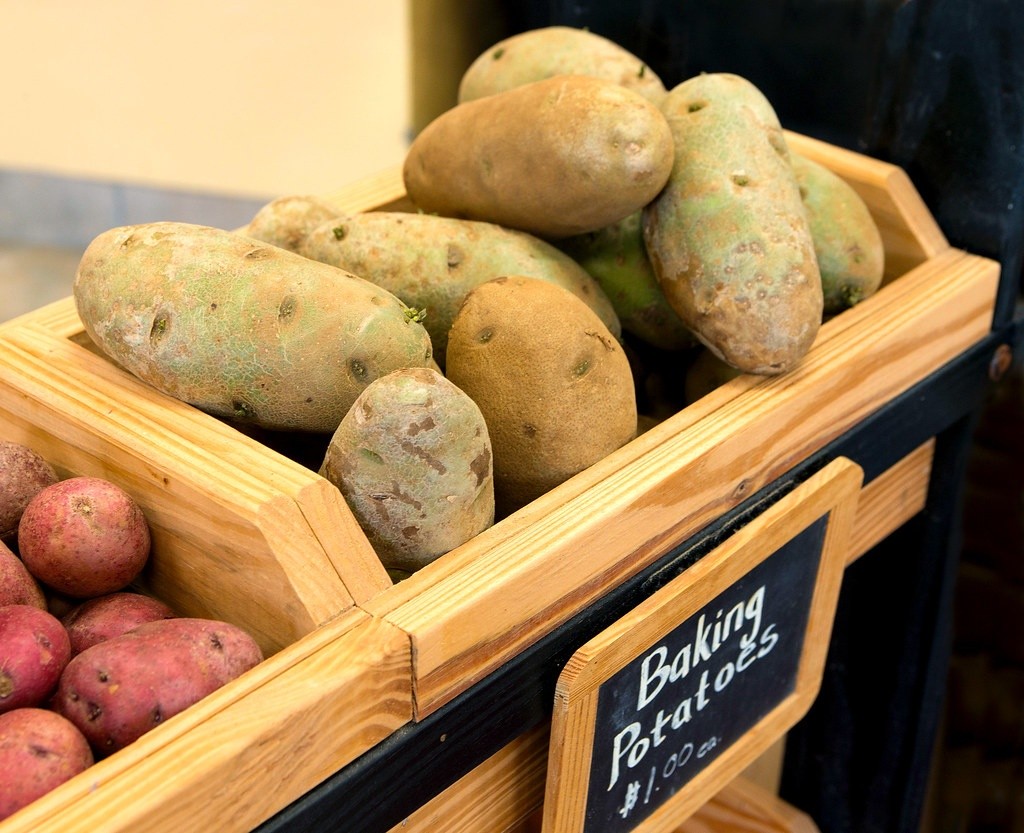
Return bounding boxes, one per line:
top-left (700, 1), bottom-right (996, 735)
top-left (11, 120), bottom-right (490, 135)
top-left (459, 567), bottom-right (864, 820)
top-left (73, 28), bottom-right (885, 581)
top-left (1, 435), bottom-right (262, 822)
